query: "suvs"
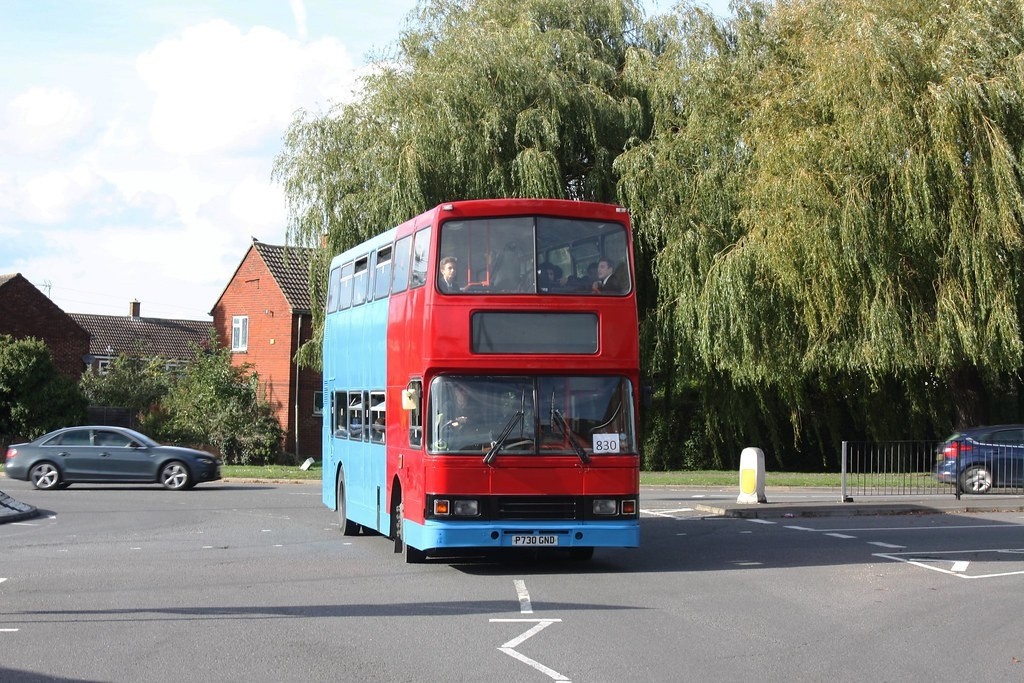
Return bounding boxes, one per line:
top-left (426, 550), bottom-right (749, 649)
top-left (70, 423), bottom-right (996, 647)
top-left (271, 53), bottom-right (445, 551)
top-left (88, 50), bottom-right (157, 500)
top-left (932, 424), bottom-right (1024, 494)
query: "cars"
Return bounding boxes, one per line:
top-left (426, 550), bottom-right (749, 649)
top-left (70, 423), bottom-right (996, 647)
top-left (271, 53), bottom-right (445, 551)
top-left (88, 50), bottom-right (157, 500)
top-left (5, 426), bottom-right (222, 492)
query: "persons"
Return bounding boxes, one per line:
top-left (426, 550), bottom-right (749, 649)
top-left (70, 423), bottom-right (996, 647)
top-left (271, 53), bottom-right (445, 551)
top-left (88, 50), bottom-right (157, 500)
top-left (592, 257), bottom-right (622, 295)
top-left (542, 263), bottom-right (566, 288)
top-left (441, 382), bottom-right (484, 438)
top-left (587, 263), bottom-right (599, 284)
top-left (375, 418), bottom-right (385, 442)
top-left (438, 257), bottom-right (461, 292)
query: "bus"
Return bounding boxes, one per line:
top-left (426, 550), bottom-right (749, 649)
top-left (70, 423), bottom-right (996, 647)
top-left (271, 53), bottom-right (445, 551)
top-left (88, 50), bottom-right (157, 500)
top-left (321, 198), bottom-right (641, 562)
